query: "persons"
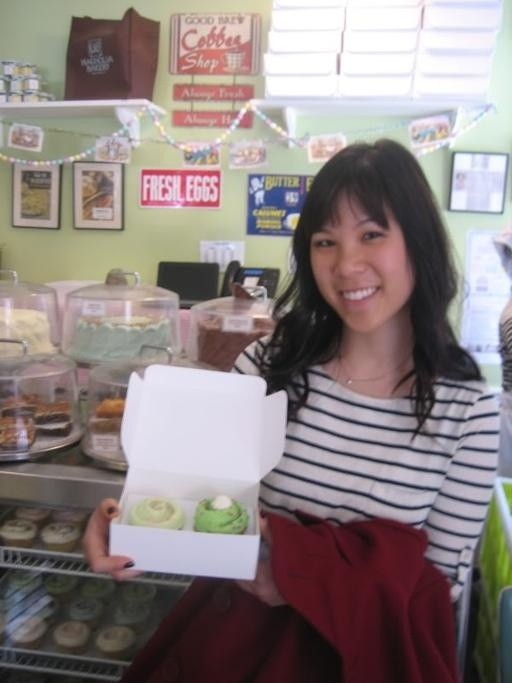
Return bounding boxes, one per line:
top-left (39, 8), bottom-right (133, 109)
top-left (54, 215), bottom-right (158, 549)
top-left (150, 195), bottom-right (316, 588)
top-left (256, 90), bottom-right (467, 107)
top-left (86, 139), bottom-right (503, 682)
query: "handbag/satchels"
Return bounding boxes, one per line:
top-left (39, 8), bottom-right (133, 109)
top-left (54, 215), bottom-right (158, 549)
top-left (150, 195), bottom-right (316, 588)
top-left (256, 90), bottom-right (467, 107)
top-left (61, 8), bottom-right (160, 99)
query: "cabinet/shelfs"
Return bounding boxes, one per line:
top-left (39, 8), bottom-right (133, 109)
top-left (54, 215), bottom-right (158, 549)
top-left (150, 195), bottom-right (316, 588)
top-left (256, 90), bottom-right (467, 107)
top-left (2, 428), bottom-right (251, 678)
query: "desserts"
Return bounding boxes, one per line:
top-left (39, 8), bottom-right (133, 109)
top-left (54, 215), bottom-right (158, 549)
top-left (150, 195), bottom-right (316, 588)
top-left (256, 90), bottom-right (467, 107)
top-left (0, 303), bottom-right (281, 451)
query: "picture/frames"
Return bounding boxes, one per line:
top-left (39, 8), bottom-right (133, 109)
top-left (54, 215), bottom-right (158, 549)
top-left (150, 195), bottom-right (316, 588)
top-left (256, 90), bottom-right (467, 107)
top-left (448, 150), bottom-right (510, 215)
top-left (72, 160), bottom-right (124, 231)
top-left (12, 160), bottom-right (62, 231)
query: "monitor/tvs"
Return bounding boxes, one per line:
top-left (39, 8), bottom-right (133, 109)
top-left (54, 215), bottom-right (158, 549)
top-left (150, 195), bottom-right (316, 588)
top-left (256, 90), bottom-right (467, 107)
top-left (157, 261), bottom-right (219, 308)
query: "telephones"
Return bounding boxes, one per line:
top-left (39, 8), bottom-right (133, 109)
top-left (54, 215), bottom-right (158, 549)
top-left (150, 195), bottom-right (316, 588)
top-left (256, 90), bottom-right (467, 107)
top-left (219, 261), bottom-right (279, 297)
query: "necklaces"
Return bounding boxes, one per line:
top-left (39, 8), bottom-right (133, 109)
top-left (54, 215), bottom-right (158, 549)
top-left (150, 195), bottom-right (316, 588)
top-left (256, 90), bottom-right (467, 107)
top-left (337, 339), bottom-right (418, 383)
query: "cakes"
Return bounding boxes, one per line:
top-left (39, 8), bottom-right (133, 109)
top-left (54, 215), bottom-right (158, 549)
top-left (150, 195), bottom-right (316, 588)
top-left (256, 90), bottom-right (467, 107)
top-left (195, 495), bottom-right (248, 535)
top-left (128, 497), bottom-right (187, 528)
top-left (0, 506), bottom-right (157, 657)
